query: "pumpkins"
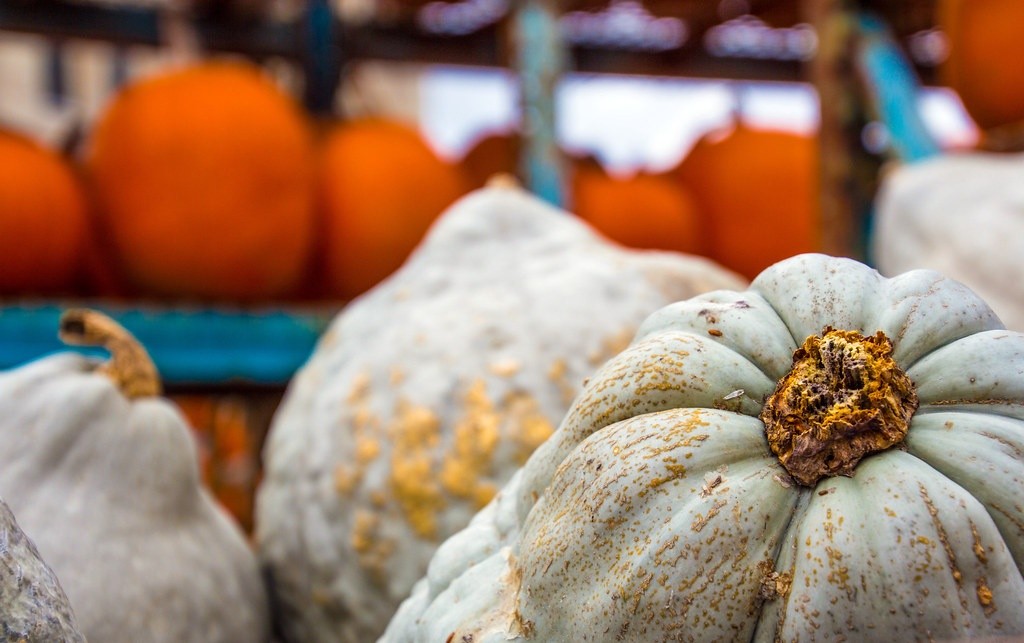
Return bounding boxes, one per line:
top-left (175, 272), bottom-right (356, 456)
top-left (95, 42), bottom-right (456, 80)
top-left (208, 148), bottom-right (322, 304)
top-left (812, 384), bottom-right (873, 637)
top-left (0, 0), bottom-right (1024, 643)
